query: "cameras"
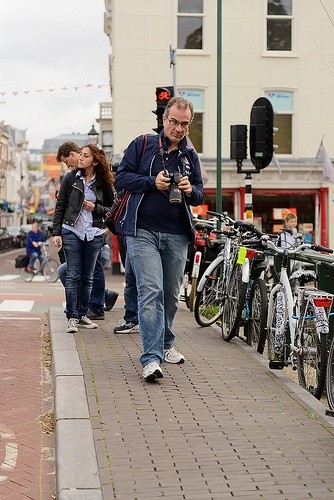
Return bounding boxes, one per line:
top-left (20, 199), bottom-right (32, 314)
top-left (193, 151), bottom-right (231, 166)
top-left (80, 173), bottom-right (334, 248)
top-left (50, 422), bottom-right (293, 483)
top-left (164, 170), bottom-right (184, 204)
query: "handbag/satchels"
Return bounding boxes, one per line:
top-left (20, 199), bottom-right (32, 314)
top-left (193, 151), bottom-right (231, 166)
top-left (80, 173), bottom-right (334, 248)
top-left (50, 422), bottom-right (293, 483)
top-left (16, 255), bottom-right (29, 267)
top-left (106, 133), bottom-right (148, 238)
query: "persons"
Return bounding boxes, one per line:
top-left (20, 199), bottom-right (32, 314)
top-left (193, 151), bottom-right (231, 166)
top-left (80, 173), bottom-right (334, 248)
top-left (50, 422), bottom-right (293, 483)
top-left (26, 223), bottom-right (48, 275)
top-left (51, 141), bottom-right (140, 333)
top-left (276, 212), bottom-right (304, 292)
top-left (115, 96), bottom-right (204, 380)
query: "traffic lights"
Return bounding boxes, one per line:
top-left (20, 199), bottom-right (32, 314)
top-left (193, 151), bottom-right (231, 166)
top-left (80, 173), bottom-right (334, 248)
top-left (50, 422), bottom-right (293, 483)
top-left (152, 86), bottom-right (175, 134)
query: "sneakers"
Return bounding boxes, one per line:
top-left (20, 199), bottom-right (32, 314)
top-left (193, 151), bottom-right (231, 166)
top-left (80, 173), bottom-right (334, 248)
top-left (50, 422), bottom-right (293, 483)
top-left (163, 347), bottom-right (185, 364)
top-left (114, 319), bottom-right (140, 333)
top-left (142, 362), bottom-right (164, 380)
top-left (77, 315), bottom-right (98, 328)
top-left (67, 318), bottom-right (78, 333)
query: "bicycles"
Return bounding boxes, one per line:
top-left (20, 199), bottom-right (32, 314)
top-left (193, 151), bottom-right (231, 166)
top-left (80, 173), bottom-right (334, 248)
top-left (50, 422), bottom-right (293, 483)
top-left (185, 210), bottom-right (334, 418)
top-left (18, 241), bottom-right (62, 283)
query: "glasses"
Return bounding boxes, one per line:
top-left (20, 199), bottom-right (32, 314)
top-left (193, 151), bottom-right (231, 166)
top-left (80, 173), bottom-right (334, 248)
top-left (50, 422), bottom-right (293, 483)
top-left (166, 114), bottom-right (192, 128)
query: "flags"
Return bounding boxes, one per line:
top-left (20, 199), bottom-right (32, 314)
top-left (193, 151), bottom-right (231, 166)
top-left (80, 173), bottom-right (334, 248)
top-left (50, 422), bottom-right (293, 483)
top-left (316, 143), bottom-right (334, 183)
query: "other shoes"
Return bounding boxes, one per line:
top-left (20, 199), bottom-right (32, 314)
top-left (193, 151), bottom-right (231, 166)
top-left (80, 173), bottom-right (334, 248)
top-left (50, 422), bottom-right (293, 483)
top-left (25, 268), bottom-right (32, 273)
top-left (104, 292), bottom-right (119, 311)
top-left (86, 310), bottom-right (105, 319)
